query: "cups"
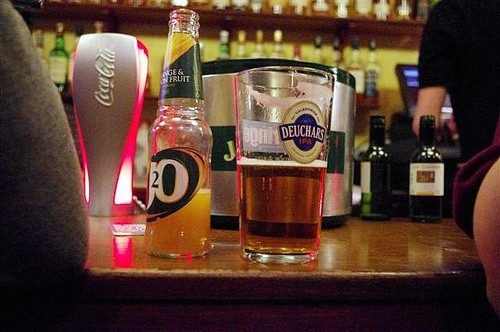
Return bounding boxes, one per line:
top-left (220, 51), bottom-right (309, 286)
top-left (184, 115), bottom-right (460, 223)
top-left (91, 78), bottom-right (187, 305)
top-left (234, 66), bottom-right (335, 265)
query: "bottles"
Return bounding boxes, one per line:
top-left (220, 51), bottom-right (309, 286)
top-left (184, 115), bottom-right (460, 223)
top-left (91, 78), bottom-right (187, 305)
top-left (416, 0), bottom-right (428, 22)
top-left (269, 29), bottom-right (284, 59)
top-left (294, 44), bottom-right (301, 61)
top-left (332, 36), bottom-right (342, 66)
top-left (35, 28), bottom-right (48, 77)
top-left (349, 37), bottom-right (365, 109)
top-left (143, 9), bottom-right (213, 258)
top-left (47, 22), bottom-right (69, 84)
top-left (314, 35), bottom-right (322, 65)
top-left (71, 24), bottom-right (83, 52)
top-left (365, 40), bottom-right (379, 108)
top-left (219, 29), bottom-right (230, 60)
top-left (236, 29), bottom-right (246, 59)
top-left (395, 0), bottom-right (414, 21)
top-left (89, 1), bottom-right (349, 16)
top-left (94, 22), bottom-right (103, 32)
top-left (408, 115), bottom-right (444, 222)
top-left (354, 0), bottom-right (393, 21)
top-left (360, 115), bottom-right (393, 220)
top-left (250, 30), bottom-right (266, 58)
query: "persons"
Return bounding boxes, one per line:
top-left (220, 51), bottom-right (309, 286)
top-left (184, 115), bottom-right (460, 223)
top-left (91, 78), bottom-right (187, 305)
top-left (0, 0), bottom-right (88, 286)
top-left (413, 0), bottom-right (499, 160)
top-left (454, 122), bottom-right (500, 314)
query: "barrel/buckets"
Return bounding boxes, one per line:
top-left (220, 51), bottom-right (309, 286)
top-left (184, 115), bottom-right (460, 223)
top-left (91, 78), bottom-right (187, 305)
top-left (199, 58), bottom-right (357, 228)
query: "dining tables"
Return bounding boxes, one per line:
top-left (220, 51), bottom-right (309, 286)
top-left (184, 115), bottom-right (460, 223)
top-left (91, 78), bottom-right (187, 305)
top-left (0, 214), bottom-right (492, 332)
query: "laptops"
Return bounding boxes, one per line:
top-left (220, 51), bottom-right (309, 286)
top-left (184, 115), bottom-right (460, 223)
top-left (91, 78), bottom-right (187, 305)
top-left (396, 64), bottom-right (454, 121)
